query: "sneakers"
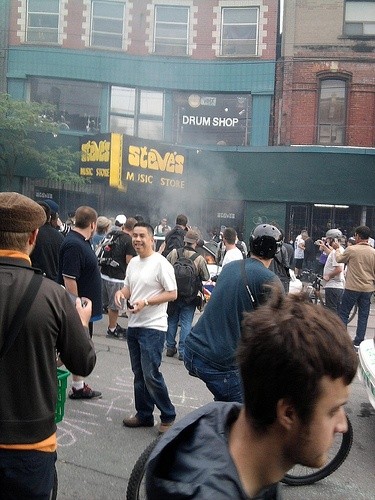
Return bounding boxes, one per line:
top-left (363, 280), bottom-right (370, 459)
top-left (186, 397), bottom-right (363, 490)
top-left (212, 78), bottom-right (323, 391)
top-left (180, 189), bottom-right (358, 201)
top-left (69, 382), bottom-right (102, 399)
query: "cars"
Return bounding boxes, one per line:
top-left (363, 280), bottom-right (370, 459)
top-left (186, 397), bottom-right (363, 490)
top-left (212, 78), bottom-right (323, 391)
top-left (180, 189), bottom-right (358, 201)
top-left (153, 234), bottom-right (222, 286)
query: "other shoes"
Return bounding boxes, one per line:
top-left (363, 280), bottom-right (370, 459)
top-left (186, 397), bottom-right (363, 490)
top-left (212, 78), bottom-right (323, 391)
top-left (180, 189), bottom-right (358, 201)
top-left (296, 274), bottom-right (302, 278)
top-left (167, 347), bottom-right (177, 357)
top-left (351, 340), bottom-right (360, 345)
top-left (179, 355), bottom-right (183, 360)
top-left (158, 422), bottom-right (174, 434)
top-left (106, 324), bottom-right (126, 340)
top-left (123, 413), bottom-right (154, 427)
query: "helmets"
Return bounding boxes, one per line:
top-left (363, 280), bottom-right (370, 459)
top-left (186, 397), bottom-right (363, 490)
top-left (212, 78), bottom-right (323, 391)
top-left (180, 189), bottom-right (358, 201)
top-left (326, 228), bottom-right (343, 239)
top-left (249, 224), bottom-right (281, 254)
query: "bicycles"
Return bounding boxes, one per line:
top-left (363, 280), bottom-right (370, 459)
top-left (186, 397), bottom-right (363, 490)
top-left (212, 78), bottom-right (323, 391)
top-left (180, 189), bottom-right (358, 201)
top-left (126, 358), bottom-right (355, 500)
top-left (299, 268), bottom-right (358, 325)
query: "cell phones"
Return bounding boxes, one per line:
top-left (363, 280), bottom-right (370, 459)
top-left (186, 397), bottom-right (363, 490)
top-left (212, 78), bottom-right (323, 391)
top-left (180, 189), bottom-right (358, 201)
top-left (120, 299), bottom-right (127, 311)
top-left (68, 292), bottom-right (77, 306)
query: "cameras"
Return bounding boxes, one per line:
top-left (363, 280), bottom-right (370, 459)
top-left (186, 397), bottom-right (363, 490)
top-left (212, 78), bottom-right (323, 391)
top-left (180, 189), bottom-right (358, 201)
top-left (333, 238), bottom-right (338, 243)
top-left (314, 241), bottom-right (321, 245)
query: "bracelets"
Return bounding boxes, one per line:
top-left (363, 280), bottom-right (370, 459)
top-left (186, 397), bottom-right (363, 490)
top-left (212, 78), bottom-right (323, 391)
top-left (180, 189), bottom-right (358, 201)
top-left (145, 299), bottom-right (148, 305)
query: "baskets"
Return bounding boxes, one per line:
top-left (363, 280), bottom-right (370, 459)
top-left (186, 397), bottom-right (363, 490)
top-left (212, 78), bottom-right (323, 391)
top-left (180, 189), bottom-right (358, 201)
top-left (55, 368), bottom-right (70, 423)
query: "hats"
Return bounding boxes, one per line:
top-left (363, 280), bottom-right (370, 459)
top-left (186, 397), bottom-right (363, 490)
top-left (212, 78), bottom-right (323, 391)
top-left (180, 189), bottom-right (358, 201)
top-left (185, 230), bottom-right (199, 243)
top-left (97, 215), bottom-right (110, 228)
top-left (116, 214), bottom-right (127, 225)
top-left (0, 192), bottom-right (46, 232)
top-left (44, 199), bottom-right (59, 210)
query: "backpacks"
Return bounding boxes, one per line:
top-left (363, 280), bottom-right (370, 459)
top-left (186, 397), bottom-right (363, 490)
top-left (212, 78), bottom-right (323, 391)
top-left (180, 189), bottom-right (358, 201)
top-left (161, 230), bottom-right (187, 257)
top-left (172, 248), bottom-right (200, 306)
top-left (97, 232), bottom-right (129, 270)
top-left (224, 241), bottom-right (246, 259)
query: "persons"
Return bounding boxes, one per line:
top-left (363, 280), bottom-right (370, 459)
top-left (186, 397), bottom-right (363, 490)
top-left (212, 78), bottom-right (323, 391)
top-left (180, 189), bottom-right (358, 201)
top-left (185, 223), bottom-right (285, 403)
top-left (29, 200), bottom-right (145, 398)
top-left (0, 192), bottom-right (97, 500)
top-left (115, 222), bottom-right (177, 432)
top-left (146, 281), bottom-right (359, 500)
top-left (154, 214), bottom-right (248, 359)
top-left (269, 226), bottom-right (375, 347)
